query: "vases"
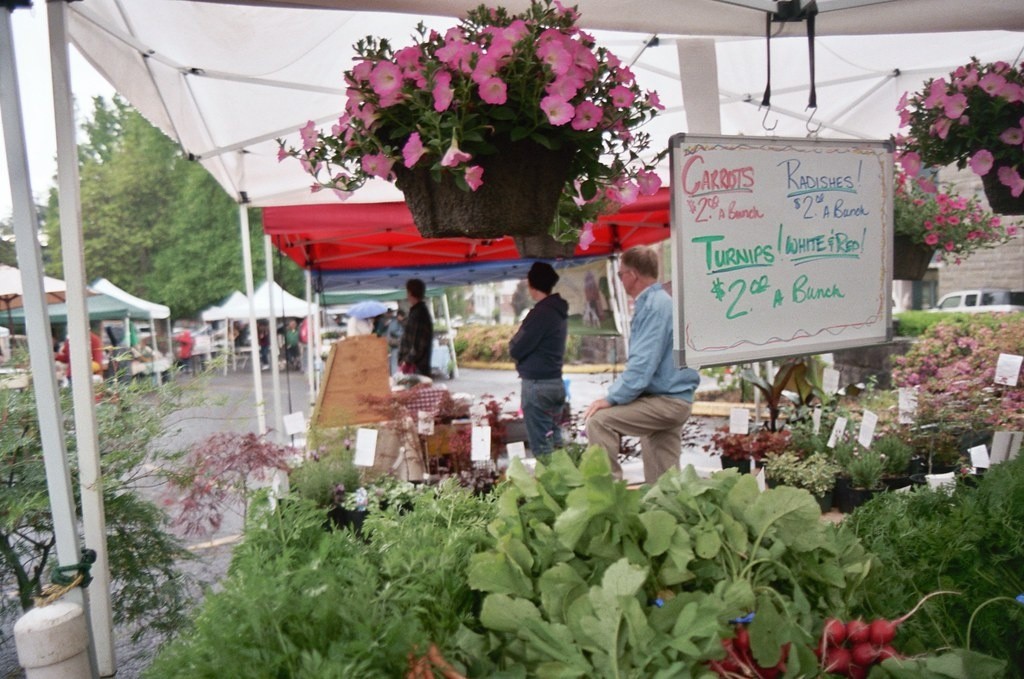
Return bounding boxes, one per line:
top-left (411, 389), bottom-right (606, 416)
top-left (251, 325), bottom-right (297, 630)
top-left (719, 454), bottom-right (763, 477)
top-left (513, 233), bottom-right (578, 261)
top-left (390, 151), bottom-right (568, 237)
top-left (893, 234), bottom-right (934, 280)
top-left (981, 164), bottom-right (1024, 215)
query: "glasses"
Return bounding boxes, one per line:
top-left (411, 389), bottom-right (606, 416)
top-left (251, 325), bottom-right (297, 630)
top-left (618, 271), bottom-right (630, 280)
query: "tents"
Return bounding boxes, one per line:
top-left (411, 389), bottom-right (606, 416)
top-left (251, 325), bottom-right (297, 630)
top-left (0, 0), bottom-right (1024, 676)
top-left (202, 280), bottom-right (325, 375)
top-left (0, 278), bottom-right (174, 391)
top-left (260, 187), bottom-right (676, 431)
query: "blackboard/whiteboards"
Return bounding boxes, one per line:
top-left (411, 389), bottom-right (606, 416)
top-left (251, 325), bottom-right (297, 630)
top-left (668, 128), bottom-right (899, 371)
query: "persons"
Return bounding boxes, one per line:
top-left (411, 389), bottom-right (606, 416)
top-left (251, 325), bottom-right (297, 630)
top-left (377, 308), bottom-right (395, 337)
top-left (261, 319), bottom-right (270, 370)
top-left (54, 323), bottom-right (101, 384)
top-left (397, 279), bottom-right (433, 377)
top-left (509, 261), bottom-right (569, 457)
top-left (584, 245), bottom-right (699, 484)
top-left (175, 331), bottom-right (193, 372)
top-left (346, 317), bottom-right (374, 337)
top-left (383, 311), bottom-right (405, 375)
top-left (286, 319), bottom-right (301, 366)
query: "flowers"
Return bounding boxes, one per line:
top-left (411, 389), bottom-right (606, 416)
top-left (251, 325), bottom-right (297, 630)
top-left (894, 54), bottom-right (1024, 197)
top-left (701, 424), bottom-right (790, 460)
top-left (550, 131), bottom-right (668, 251)
top-left (271, 0), bottom-right (668, 202)
top-left (891, 162), bottom-right (1023, 264)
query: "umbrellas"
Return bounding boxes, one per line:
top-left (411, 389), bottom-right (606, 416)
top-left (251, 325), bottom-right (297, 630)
top-left (344, 299), bottom-right (388, 319)
top-left (0, 262), bottom-right (97, 348)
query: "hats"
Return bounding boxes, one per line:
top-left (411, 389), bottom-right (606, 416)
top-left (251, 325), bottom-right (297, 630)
top-left (530, 262), bottom-right (560, 288)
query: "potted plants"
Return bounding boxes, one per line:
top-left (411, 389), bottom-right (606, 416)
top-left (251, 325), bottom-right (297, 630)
top-left (759, 427), bottom-right (993, 514)
